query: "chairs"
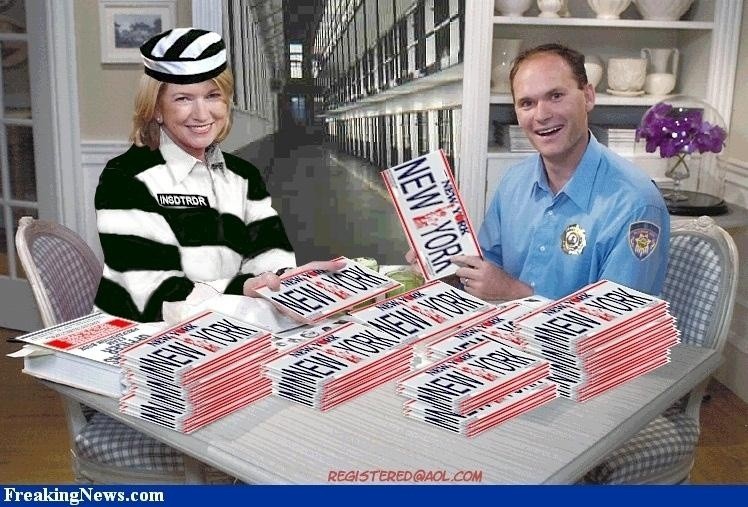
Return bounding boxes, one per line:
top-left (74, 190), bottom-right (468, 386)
top-left (14, 214), bottom-right (240, 486)
top-left (567, 214), bottom-right (741, 484)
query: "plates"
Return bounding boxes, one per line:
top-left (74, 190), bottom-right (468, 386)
top-left (605, 88), bottom-right (645, 97)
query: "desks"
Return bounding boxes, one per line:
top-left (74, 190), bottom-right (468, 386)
top-left (22, 296), bottom-right (727, 485)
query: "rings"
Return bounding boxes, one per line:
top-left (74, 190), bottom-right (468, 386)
top-left (464, 279), bottom-right (469, 286)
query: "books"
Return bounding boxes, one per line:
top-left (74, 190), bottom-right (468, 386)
top-left (6, 312), bottom-right (159, 399)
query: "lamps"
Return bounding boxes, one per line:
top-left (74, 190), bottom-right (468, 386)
top-left (634, 94), bottom-right (730, 216)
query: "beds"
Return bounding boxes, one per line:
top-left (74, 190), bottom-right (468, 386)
top-left (658, 188), bottom-right (728, 218)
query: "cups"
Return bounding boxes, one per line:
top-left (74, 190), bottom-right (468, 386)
top-left (606, 57), bottom-right (648, 92)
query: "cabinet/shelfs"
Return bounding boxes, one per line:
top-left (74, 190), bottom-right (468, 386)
top-left (460, 1), bottom-right (748, 243)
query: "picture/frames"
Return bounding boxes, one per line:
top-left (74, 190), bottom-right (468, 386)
top-left (97, 0), bottom-right (178, 66)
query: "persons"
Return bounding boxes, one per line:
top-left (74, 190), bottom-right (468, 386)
top-left (89, 28), bottom-right (348, 327)
top-left (404, 44), bottom-right (670, 303)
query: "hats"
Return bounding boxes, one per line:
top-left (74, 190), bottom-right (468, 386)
top-left (140, 28), bottom-right (226, 83)
